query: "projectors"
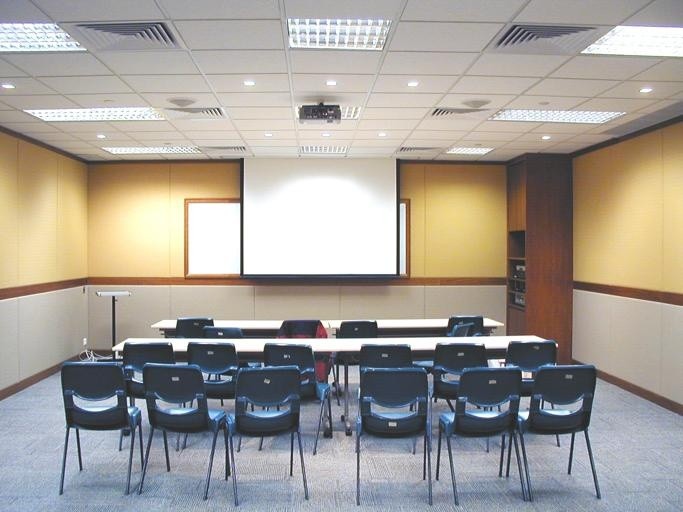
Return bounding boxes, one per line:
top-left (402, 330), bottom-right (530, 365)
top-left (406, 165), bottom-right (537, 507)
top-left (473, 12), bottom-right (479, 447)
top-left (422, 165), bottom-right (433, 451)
top-left (298, 105), bottom-right (342, 124)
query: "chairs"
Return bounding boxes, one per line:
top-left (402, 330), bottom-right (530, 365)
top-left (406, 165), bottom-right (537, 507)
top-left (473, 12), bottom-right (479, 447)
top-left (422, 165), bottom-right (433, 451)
top-left (109, 316), bottom-right (562, 455)
top-left (58, 363), bottom-right (143, 495)
top-left (138, 363), bottom-right (229, 501)
top-left (355, 367), bottom-right (434, 507)
top-left (438, 365), bottom-right (528, 505)
top-left (226, 365), bottom-right (310, 506)
top-left (507, 364), bottom-right (600, 502)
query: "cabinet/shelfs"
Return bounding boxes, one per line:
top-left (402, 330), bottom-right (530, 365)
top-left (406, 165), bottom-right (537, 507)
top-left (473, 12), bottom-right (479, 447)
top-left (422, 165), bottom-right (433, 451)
top-left (505, 152), bottom-right (572, 365)
